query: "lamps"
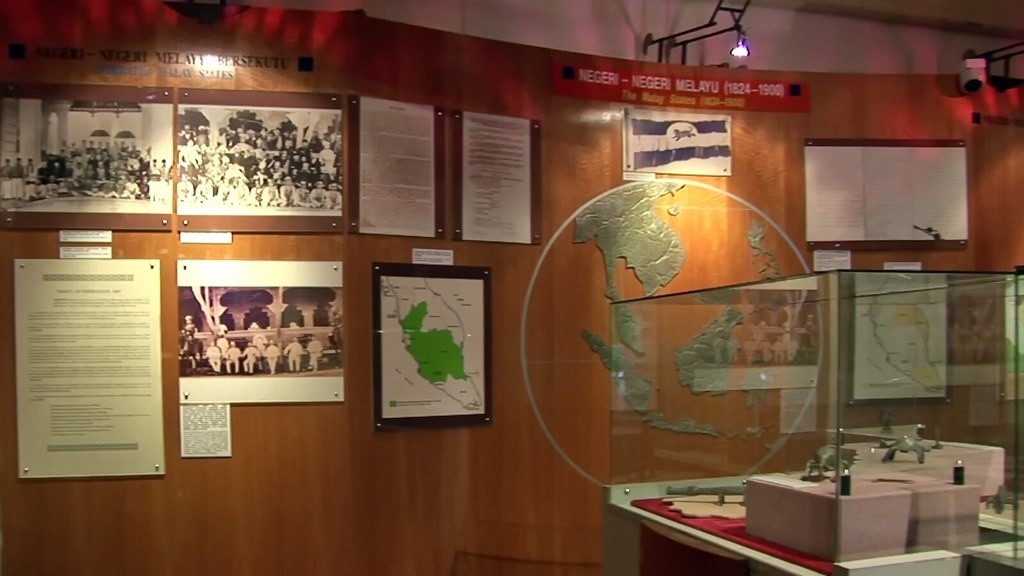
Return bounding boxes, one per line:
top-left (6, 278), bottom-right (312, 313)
top-left (730, 28), bottom-right (750, 57)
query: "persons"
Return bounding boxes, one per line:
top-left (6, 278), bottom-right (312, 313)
top-left (0, 139), bottom-right (168, 202)
top-left (180, 315), bottom-right (322, 376)
top-left (176, 124), bottom-right (342, 211)
